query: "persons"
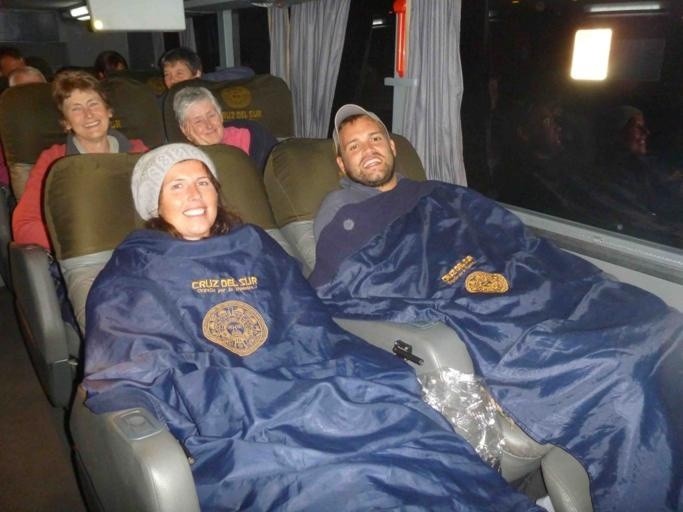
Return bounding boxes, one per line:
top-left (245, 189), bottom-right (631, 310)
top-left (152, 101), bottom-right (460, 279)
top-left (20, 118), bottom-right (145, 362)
top-left (13, 68), bottom-right (151, 341)
top-left (94, 50), bottom-right (128, 80)
top-left (315, 104), bottom-right (683, 511)
top-left (0, 50), bottom-right (26, 89)
top-left (130, 143), bottom-right (547, 511)
top-left (171, 85), bottom-right (280, 180)
top-left (144, 47), bottom-right (255, 89)
top-left (0, 66), bottom-right (49, 187)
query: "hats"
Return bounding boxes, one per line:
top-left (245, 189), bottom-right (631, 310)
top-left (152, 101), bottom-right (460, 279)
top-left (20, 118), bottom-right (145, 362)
top-left (332, 103), bottom-right (389, 157)
top-left (130, 141), bottom-right (218, 221)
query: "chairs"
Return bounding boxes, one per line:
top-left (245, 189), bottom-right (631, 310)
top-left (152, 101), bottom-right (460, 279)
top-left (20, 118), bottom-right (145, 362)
top-left (0, 55), bottom-right (596, 512)
